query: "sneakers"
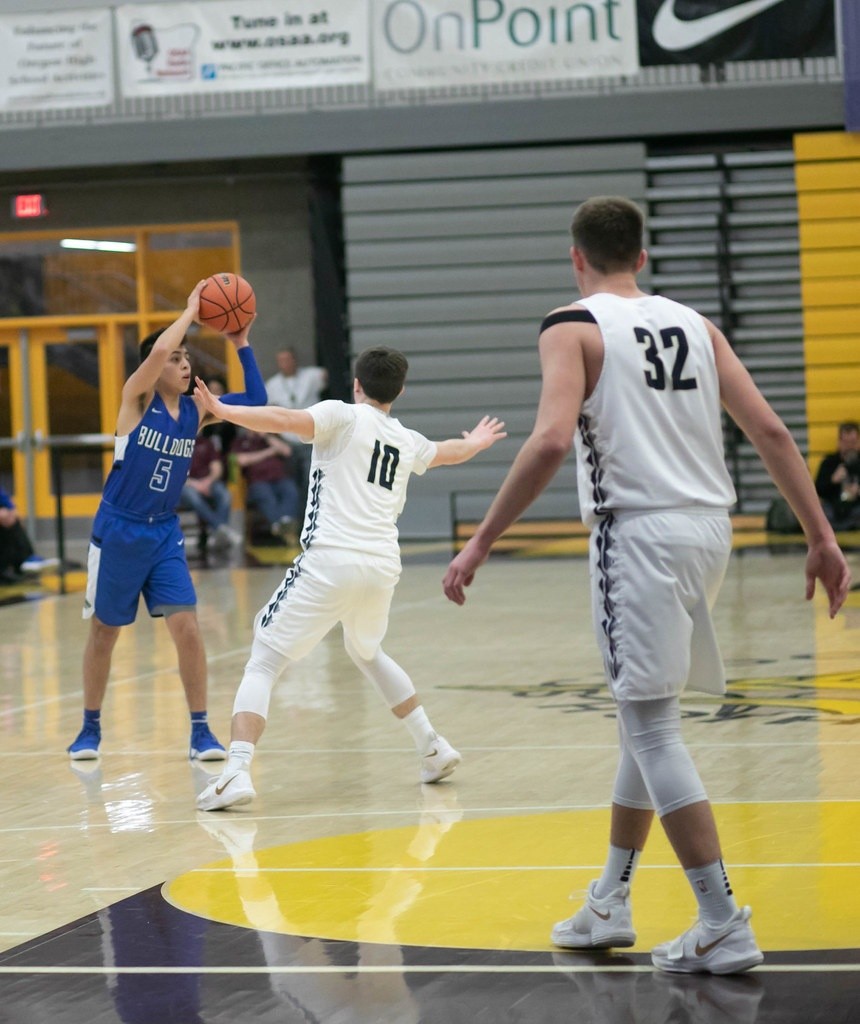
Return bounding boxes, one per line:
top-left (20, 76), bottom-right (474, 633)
top-left (67, 719), bottom-right (101, 760)
top-left (189, 724), bottom-right (228, 760)
top-left (72, 760), bottom-right (103, 784)
top-left (420, 783), bottom-right (464, 834)
top-left (194, 769), bottom-right (256, 811)
top-left (197, 810), bottom-right (258, 852)
top-left (420, 731), bottom-right (461, 783)
top-left (20, 555), bottom-right (60, 572)
top-left (188, 760), bottom-right (224, 793)
top-left (552, 879), bottom-right (636, 947)
top-left (653, 972), bottom-right (761, 1024)
top-left (552, 952), bottom-right (635, 1011)
top-left (652, 906), bottom-right (764, 974)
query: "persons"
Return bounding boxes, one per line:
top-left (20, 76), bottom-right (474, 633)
top-left (67, 280), bottom-right (268, 760)
top-left (815, 423), bottom-right (860, 532)
top-left (442, 197), bottom-right (851, 974)
top-left (182, 348), bottom-right (330, 565)
top-left (194, 346), bottom-right (507, 810)
top-left (0, 485), bottom-right (60, 573)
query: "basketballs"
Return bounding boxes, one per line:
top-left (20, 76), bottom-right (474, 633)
top-left (199, 272), bottom-right (257, 332)
